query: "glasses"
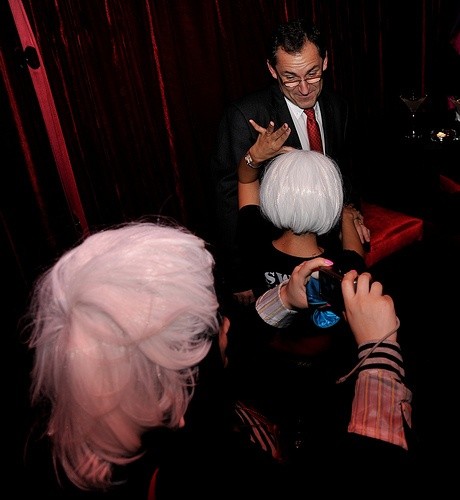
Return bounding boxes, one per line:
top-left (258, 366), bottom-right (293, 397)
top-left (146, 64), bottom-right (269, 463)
top-left (273, 59), bottom-right (325, 87)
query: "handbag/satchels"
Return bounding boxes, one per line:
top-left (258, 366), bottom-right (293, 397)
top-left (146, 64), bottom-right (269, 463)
top-left (230, 399), bottom-right (305, 463)
top-left (356, 202), bottom-right (425, 271)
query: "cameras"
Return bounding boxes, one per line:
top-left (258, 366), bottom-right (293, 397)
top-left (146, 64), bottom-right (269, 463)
top-left (319, 269), bottom-right (357, 310)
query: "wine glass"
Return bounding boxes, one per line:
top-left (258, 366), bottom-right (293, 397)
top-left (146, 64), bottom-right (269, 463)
top-left (400, 92), bottom-right (428, 139)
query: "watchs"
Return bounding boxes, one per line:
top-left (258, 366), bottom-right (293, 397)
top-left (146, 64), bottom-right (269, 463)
top-left (244, 150), bottom-right (263, 169)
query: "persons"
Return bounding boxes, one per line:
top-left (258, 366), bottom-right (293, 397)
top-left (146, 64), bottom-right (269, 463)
top-left (218, 21), bottom-right (371, 356)
top-left (28, 218), bottom-right (412, 499)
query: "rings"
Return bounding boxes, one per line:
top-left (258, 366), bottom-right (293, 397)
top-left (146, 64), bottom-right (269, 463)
top-left (355, 216), bottom-right (361, 223)
